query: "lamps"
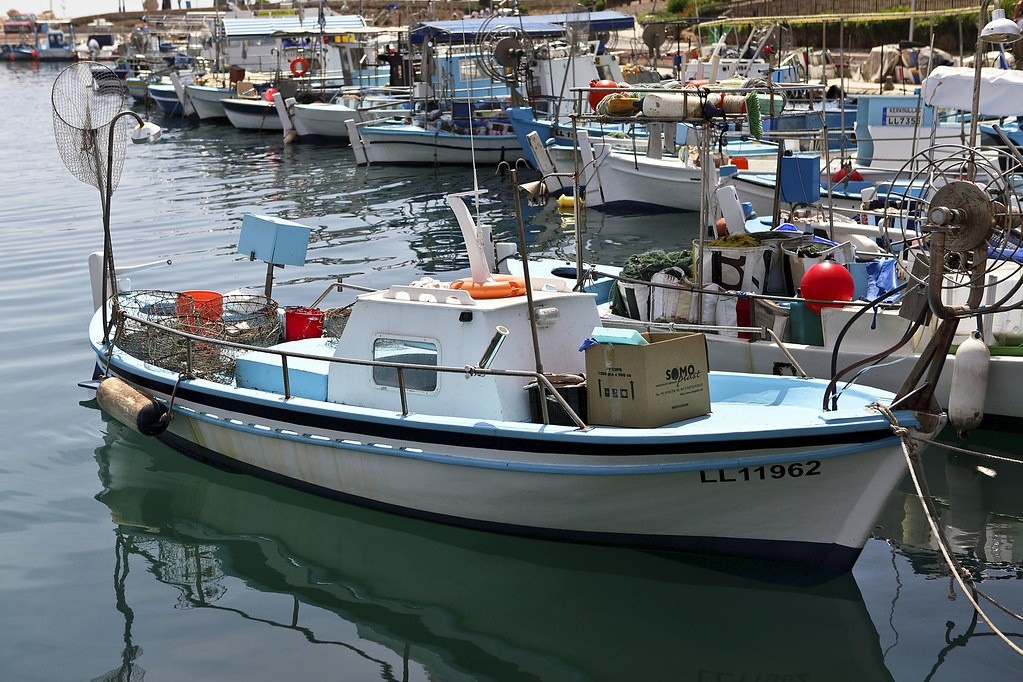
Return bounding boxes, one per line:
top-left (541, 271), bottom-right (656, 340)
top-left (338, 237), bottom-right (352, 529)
top-left (979, 10), bottom-right (1021, 44)
top-left (131, 122), bottom-right (162, 144)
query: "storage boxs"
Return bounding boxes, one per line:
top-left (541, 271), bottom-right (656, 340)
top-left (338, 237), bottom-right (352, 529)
top-left (237, 214), bottom-right (310, 267)
top-left (584, 333), bottom-right (712, 429)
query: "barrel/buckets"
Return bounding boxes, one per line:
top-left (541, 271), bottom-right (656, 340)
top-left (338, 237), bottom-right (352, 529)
top-left (176, 290), bottom-right (224, 353)
top-left (286, 306), bottom-right (325, 342)
top-left (730, 157), bottom-right (748, 170)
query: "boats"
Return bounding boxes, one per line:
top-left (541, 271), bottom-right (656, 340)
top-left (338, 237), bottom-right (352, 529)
top-left (0, 0), bottom-right (1023, 425)
top-left (88, 419), bottom-right (893, 682)
top-left (79, 91), bottom-right (950, 588)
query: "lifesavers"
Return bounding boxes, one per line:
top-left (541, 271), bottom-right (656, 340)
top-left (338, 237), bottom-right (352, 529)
top-left (290, 57), bottom-right (308, 76)
top-left (449, 274), bottom-right (526, 299)
